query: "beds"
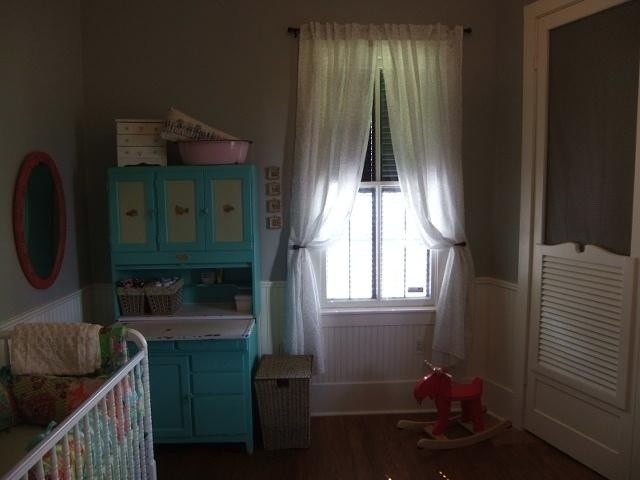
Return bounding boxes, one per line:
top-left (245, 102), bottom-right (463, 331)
top-left (1, 325), bottom-right (162, 480)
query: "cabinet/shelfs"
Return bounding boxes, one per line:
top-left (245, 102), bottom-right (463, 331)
top-left (106, 163), bottom-right (261, 320)
top-left (110, 320), bottom-right (262, 456)
top-left (114, 117), bottom-right (169, 168)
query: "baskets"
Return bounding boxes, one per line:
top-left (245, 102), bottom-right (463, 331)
top-left (142, 271), bottom-right (185, 316)
top-left (113, 275), bottom-right (152, 318)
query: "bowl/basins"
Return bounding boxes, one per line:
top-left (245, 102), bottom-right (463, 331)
top-left (174, 138), bottom-right (254, 165)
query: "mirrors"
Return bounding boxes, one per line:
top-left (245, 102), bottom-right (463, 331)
top-left (14, 151), bottom-right (69, 290)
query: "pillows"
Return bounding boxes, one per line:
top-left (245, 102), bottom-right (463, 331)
top-left (1, 372), bottom-right (107, 433)
